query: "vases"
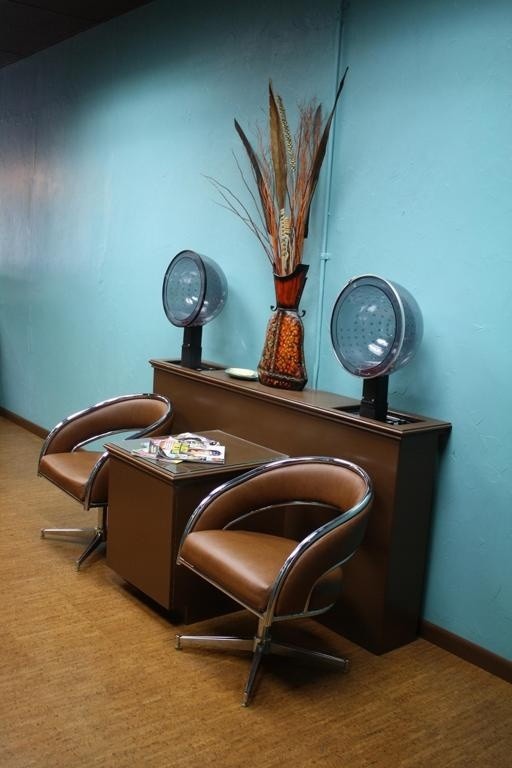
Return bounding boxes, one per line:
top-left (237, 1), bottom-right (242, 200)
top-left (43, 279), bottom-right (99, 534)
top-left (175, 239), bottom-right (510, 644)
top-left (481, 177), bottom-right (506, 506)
top-left (257, 264), bottom-right (309, 391)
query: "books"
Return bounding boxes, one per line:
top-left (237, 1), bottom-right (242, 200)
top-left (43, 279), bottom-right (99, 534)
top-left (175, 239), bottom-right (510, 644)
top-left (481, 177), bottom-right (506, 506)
top-left (132, 432), bottom-right (226, 465)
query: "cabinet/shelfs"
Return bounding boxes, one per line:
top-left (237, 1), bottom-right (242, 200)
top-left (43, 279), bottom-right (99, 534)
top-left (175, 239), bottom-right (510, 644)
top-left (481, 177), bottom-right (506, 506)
top-left (149, 359), bottom-right (452, 656)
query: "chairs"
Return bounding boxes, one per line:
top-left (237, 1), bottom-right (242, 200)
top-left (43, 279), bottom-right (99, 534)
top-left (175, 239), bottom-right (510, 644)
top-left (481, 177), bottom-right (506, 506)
top-left (176, 456), bottom-right (374, 705)
top-left (39, 394), bottom-right (175, 568)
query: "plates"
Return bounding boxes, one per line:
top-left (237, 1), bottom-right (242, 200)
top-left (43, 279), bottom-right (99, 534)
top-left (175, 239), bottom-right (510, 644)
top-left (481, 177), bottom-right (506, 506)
top-left (225, 368), bottom-right (258, 380)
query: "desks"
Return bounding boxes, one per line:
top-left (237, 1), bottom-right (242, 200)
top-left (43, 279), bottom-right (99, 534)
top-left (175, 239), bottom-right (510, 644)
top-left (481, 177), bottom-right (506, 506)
top-left (105, 430), bottom-right (290, 626)
